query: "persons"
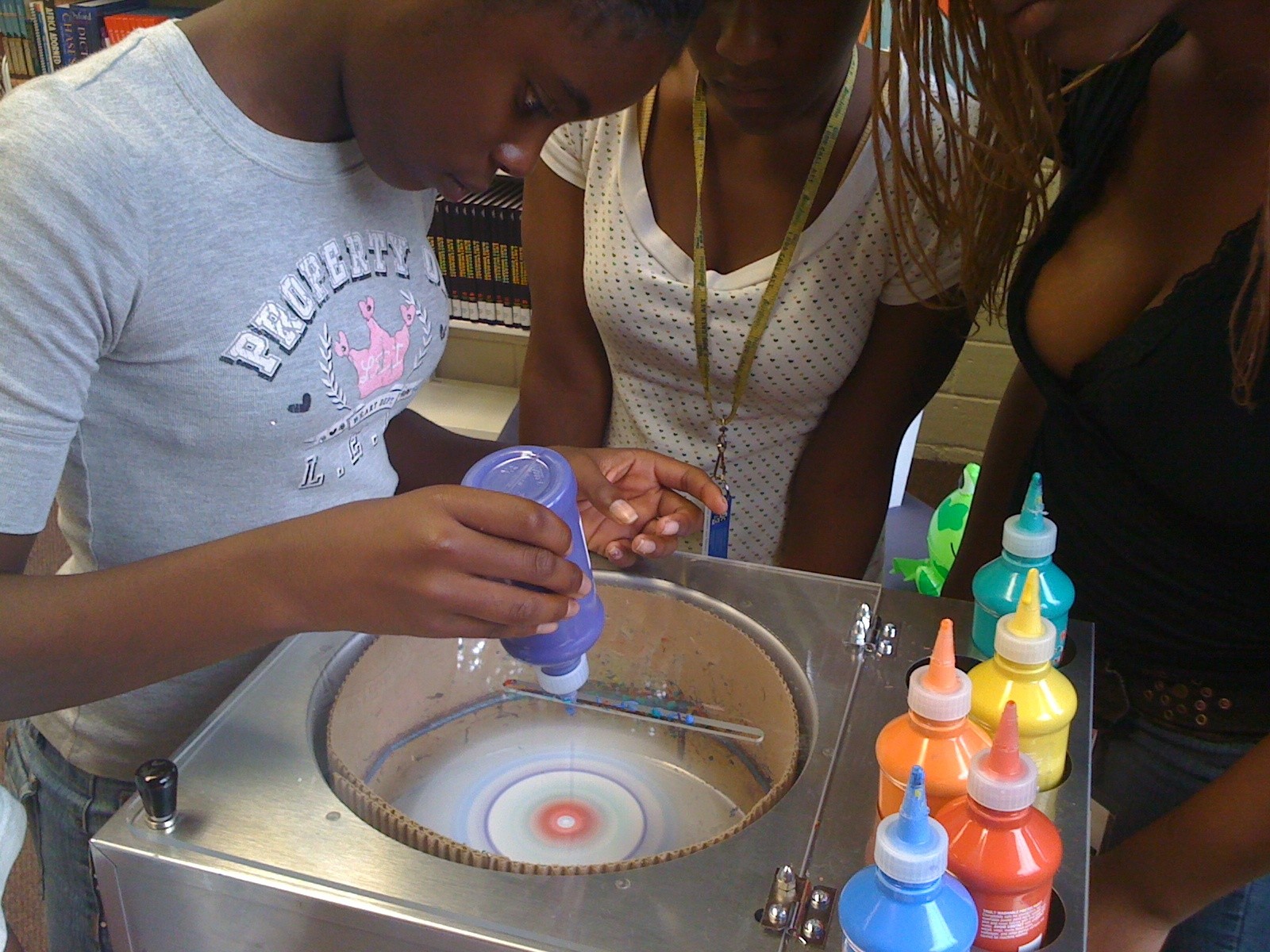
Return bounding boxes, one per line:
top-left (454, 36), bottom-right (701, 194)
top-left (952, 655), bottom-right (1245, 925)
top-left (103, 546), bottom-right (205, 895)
top-left (0, 0), bottom-right (699, 952)
top-left (491, 0), bottom-right (1270, 952)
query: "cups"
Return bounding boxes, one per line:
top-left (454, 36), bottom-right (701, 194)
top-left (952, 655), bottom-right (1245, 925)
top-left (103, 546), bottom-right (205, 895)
top-left (1031, 751), bottom-right (1073, 824)
top-left (905, 654), bottom-right (983, 691)
top-left (1036, 886), bottom-right (1068, 952)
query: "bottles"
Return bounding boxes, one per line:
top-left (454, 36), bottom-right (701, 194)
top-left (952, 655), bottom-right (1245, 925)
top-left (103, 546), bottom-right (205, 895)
top-left (968, 568), bottom-right (1078, 793)
top-left (460, 446), bottom-right (605, 716)
top-left (935, 700), bottom-right (1062, 952)
top-left (969, 472), bottom-right (1075, 669)
top-left (875, 619), bottom-right (993, 821)
top-left (838, 765), bottom-right (979, 952)
